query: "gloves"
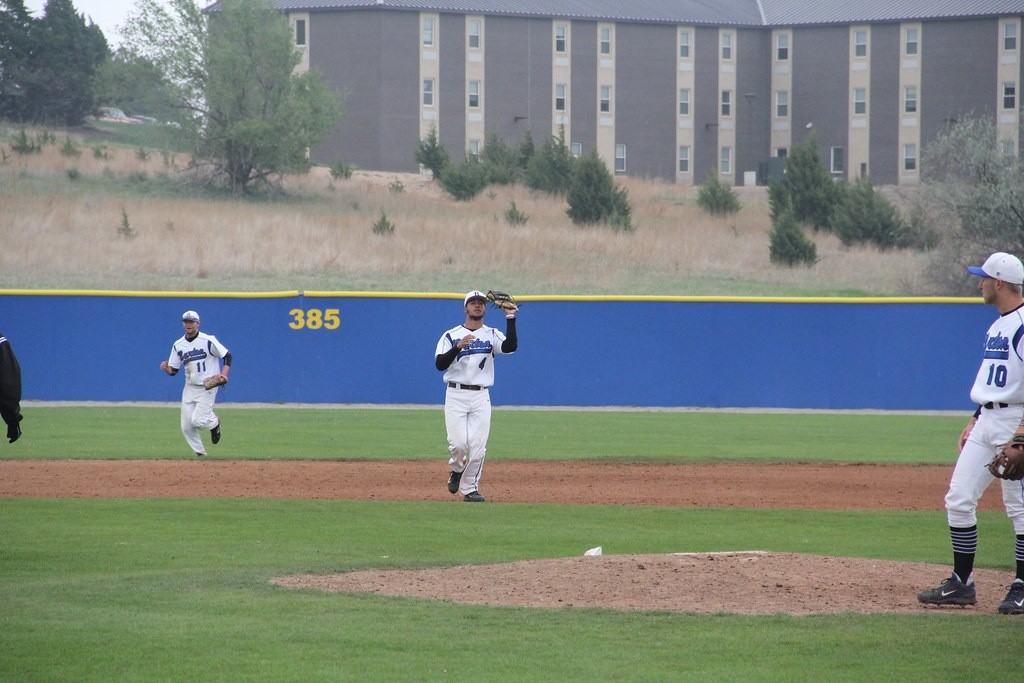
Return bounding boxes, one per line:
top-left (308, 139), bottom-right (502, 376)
top-left (7, 423), bottom-right (21, 443)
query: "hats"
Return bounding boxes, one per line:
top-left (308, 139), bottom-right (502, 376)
top-left (182, 311), bottom-right (202, 325)
top-left (966, 251), bottom-right (1023, 286)
top-left (463, 290), bottom-right (490, 305)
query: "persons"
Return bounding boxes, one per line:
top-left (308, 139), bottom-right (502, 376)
top-left (435, 290), bottom-right (519, 502)
top-left (160, 311), bottom-right (232, 456)
top-left (0, 334), bottom-right (24, 444)
top-left (916, 252), bottom-right (1024, 613)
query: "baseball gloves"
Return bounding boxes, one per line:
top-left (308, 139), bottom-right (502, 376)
top-left (983, 418), bottom-right (1024, 481)
top-left (202, 374), bottom-right (228, 390)
top-left (486, 290), bottom-right (523, 312)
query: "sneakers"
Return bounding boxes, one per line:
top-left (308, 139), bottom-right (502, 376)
top-left (210, 420), bottom-right (222, 445)
top-left (463, 490), bottom-right (487, 503)
top-left (917, 573), bottom-right (977, 605)
top-left (446, 470), bottom-right (465, 494)
top-left (997, 582), bottom-right (1024, 615)
top-left (197, 454), bottom-right (203, 458)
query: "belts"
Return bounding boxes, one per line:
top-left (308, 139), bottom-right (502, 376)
top-left (448, 382), bottom-right (489, 390)
top-left (983, 401), bottom-right (1010, 411)
